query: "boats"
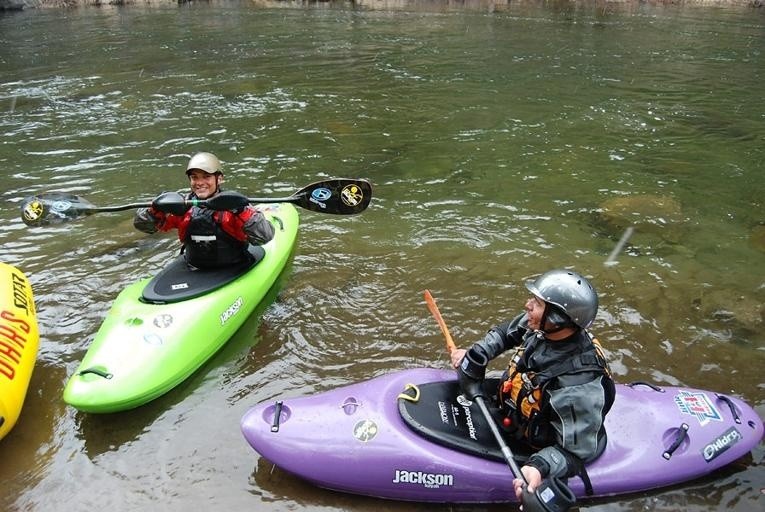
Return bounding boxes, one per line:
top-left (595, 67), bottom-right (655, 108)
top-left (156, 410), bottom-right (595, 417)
top-left (62, 198), bottom-right (300, 412)
top-left (241, 365), bottom-right (765, 505)
top-left (1, 262), bottom-right (39, 440)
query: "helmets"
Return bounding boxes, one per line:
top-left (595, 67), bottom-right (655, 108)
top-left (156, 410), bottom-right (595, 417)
top-left (525, 270), bottom-right (598, 330)
top-left (186, 152), bottom-right (223, 175)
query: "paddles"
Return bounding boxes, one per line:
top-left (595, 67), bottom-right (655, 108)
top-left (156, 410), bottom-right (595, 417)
top-left (424, 288), bottom-right (528, 488)
top-left (21, 179), bottom-right (371, 228)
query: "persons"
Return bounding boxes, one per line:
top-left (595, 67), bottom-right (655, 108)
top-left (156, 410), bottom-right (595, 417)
top-left (133, 153), bottom-right (275, 267)
top-left (450, 267), bottom-right (615, 511)
